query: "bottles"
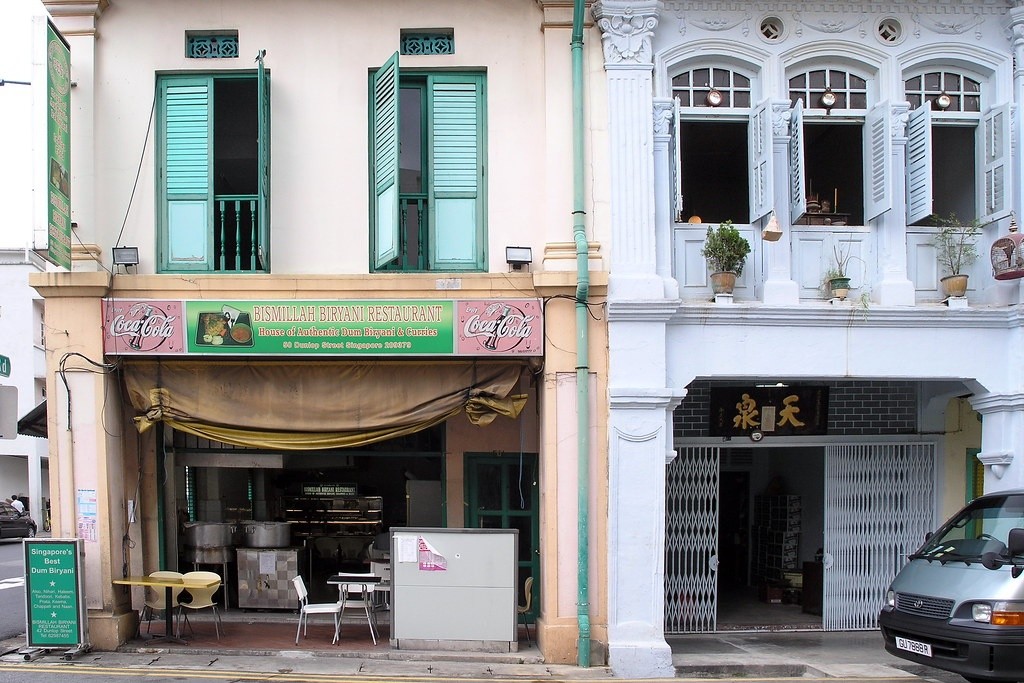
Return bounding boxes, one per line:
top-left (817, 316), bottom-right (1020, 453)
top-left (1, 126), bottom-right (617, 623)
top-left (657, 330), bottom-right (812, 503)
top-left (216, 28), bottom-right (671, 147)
top-left (771, 599), bottom-right (782, 603)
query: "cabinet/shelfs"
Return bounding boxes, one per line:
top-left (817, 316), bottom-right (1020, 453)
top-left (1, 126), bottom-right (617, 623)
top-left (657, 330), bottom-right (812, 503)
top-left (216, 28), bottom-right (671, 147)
top-left (283, 496), bottom-right (383, 536)
top-left (803, 561), bottom-right (823, 617)
top-left (795, 212), bottom-right (851, 226)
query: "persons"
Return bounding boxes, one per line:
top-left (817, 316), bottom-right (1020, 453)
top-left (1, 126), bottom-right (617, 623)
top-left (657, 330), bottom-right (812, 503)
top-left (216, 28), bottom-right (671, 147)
top-left (11, 495), bottom-right (25, 539)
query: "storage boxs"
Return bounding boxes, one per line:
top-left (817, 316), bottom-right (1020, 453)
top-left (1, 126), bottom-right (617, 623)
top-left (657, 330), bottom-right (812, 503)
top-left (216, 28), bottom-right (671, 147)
top-left (751, 495), bottom-right (802, 588)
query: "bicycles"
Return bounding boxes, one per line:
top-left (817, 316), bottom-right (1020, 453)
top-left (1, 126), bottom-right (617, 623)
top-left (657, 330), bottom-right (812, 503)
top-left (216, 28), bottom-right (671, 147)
top-left (41, 508), bottom-right (51, 532)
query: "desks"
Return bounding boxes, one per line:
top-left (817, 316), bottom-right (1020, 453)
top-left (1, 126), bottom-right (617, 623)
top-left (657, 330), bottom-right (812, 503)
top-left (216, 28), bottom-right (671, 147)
top-left (328, 575), bottom-right (382, 645)
top-left (112, 576), bottom-right (222, 646)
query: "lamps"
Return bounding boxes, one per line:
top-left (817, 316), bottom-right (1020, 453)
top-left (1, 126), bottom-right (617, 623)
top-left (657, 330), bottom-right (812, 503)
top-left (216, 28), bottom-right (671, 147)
top-left (112, 247), bottom-right (139, 274)
top-left (505, 247), bottom-right (533, 273)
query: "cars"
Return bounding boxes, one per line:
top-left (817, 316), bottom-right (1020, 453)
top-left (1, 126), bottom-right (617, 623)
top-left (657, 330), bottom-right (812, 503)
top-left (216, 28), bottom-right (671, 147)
top-left (0, 501), bottom-right (37, 538)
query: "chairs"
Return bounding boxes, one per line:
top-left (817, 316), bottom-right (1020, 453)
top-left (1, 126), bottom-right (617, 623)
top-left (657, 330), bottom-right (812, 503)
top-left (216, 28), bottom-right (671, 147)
top-left (291, 561), bottom-right (391, 645)
top-left (518, 576), bottom-right (533, 648)
top-left (134, 571), bottom-right (226, 641)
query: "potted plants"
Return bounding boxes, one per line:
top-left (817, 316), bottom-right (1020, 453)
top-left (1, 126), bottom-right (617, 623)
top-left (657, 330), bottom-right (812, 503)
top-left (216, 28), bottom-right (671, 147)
top-left (700, 220), bottom-right (752, 295)
top-left (929, 211), bottom-right (984, 298)
top-left (816, 234), bottom-right (872, 323)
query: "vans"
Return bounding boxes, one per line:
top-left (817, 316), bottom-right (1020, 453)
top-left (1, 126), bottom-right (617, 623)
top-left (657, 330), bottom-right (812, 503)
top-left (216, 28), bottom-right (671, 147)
top-left (879, 486), bottom-right (1024, 683)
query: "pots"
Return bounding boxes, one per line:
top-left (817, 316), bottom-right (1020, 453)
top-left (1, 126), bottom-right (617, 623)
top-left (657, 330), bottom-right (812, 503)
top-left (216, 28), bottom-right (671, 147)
top-left (244, 521), bottom-right (292, 548)
top-left (183, 521), bottom-right (238, 548)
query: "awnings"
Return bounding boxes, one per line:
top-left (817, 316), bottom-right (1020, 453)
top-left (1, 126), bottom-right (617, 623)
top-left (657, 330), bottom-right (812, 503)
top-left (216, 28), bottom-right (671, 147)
top-left (16, 398), bottom-right (49, 439)
